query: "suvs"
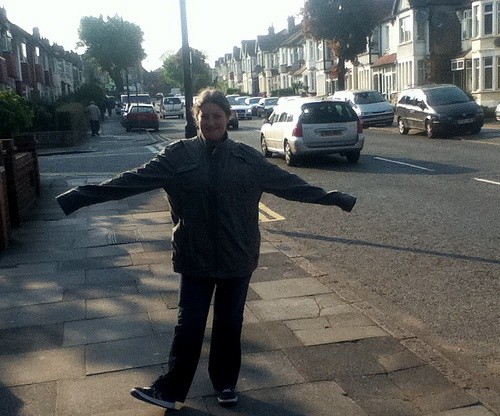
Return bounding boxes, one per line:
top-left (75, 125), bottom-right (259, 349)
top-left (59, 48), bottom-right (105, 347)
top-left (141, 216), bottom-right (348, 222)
top-left (259, 98), bottom-right (365, 166)
top-left (332, 89), bottom-right (395, 129)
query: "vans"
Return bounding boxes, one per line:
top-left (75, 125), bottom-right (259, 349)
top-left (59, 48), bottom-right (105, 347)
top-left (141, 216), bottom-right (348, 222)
top-left (392, 83), bottom-right (485, 139)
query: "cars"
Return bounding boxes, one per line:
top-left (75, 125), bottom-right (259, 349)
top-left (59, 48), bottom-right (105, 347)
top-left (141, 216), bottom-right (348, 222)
top-left (121, 102), bottom-right (160, 133)
top-left (154, 93), bottom-right (279, 129)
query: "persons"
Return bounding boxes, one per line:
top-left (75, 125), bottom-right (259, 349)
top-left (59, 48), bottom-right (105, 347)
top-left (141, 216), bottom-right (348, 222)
top-left (55, 89), bottom-right (357, 409)
top-left (104, 93), bottom-right (115, 116)
top-left (86, 100), bottom-right (101, 137)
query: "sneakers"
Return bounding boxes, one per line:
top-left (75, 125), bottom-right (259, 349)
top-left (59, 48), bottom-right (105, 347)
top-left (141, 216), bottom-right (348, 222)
top-left (130, 384), bottom-right (175, 410)
top-left (217, 387), bottom-right (239, 406)
